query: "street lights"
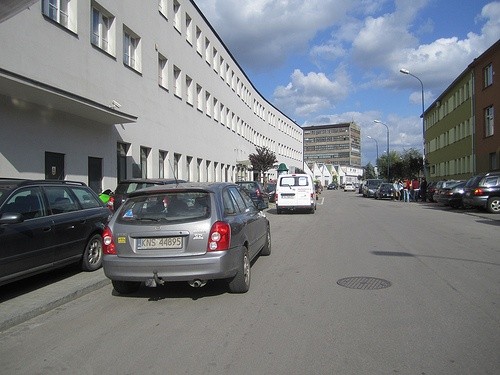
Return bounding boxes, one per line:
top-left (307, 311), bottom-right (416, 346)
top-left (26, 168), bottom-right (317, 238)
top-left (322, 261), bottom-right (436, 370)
top-left (368, 136), bottom-right (378, 158)
top-left (400, 69), bottom-right (425, 154)
top-left (373, 120), bottom-right (389, 156)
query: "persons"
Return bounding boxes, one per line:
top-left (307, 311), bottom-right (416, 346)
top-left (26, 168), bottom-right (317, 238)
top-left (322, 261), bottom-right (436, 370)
top-left (393, 177), bottom-right (427, 202)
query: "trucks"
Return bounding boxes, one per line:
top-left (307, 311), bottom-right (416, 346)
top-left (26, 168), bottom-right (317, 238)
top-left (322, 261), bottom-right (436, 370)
top-left (275, 173), bottom-right (317, 214)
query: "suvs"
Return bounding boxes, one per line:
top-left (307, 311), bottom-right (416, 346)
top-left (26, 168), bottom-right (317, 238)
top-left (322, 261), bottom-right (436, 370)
top-left (463, 171), bottom-right (500, 213)
top-left (361, 179), bottom-right (384, 196)
top-left (0, 177), bottom-right (110, 288)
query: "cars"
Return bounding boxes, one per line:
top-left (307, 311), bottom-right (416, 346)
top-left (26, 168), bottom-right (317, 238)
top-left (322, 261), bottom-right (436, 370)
top-left (344, 181), bottom-right (356, 192)
top-left (425, 181), bottom-right (436, 199)
top-left (431, 180), bottom-right (460, 202)
top-left (438, 180), bottom-right (468, 209)
top-left (101, 157), bottom-right (271, 294)
top-left (233, 180), bottom-right (270, 209)
top-left (108, 177), bottom-right (187, 212)
top-left (374, 183), bottom-right (397, 200)
top-left (327, 184), bottom-right (338, 189)
